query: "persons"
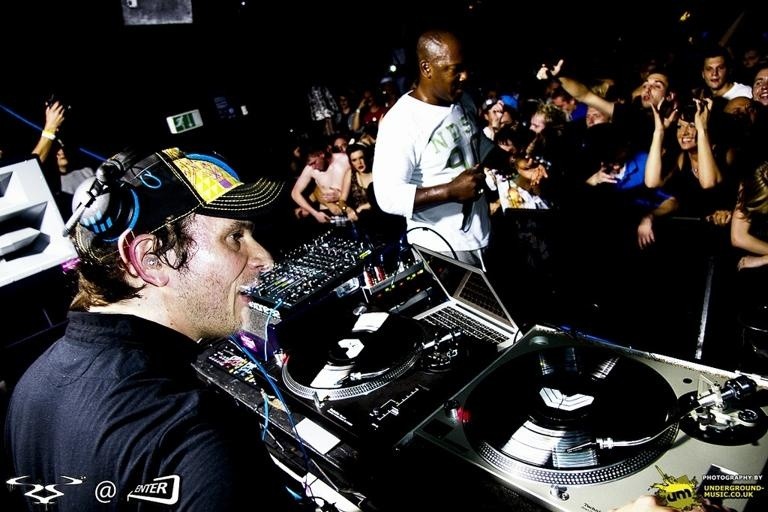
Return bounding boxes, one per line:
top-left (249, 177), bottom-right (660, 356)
top-left (529, 105), bottom-right (566, 133)
top-left (582, 107), bottom-right (612, 129)
top-left (750, 65), bottom-right (768, 108)
top-left (551, 86), bottom-right (586, 120)
top-left (719, 97), bottom-right (767, 163)
top-left (478, 97), bottom-right (506, 142)
top-left (645, 93), bottom-right (735, 188)
top-left (289, 137), bottom-right (351, 223)
top-left (731, 163), bottom-right (768, 273)
top-left (352, 89), bottom-right (386, 131)
top-left (373, 32), bottom-right (547, 304)
top-left (339, 142), bottom-right (379, 222)
top-left (537, 58), bottom-right (679, 167)
top-left (492, 125), bottom-right (524, 155)
top-left (27, 100), bottom-right (95, 217)
top-left (567, 124), bottom-right (679, 248)
top-left (330, 93), bottom-right (357, 133)
top-left (702, 45), bottom-right (753, 102)
top-left (1, 147), bottom-right (287, 512)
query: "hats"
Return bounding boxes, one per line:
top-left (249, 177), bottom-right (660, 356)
top-left (61, 147), bottom-right (288, 277)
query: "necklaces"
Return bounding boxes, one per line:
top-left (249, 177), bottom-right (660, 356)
top-left (688, 162), bottom-right (701, 176)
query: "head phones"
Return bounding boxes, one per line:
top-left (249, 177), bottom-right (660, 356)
top-left (62, 139), bottom-right (161, 244)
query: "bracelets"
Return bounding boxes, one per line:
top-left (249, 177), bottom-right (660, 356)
top-left (40, 130), bottom-right (57, 141)
